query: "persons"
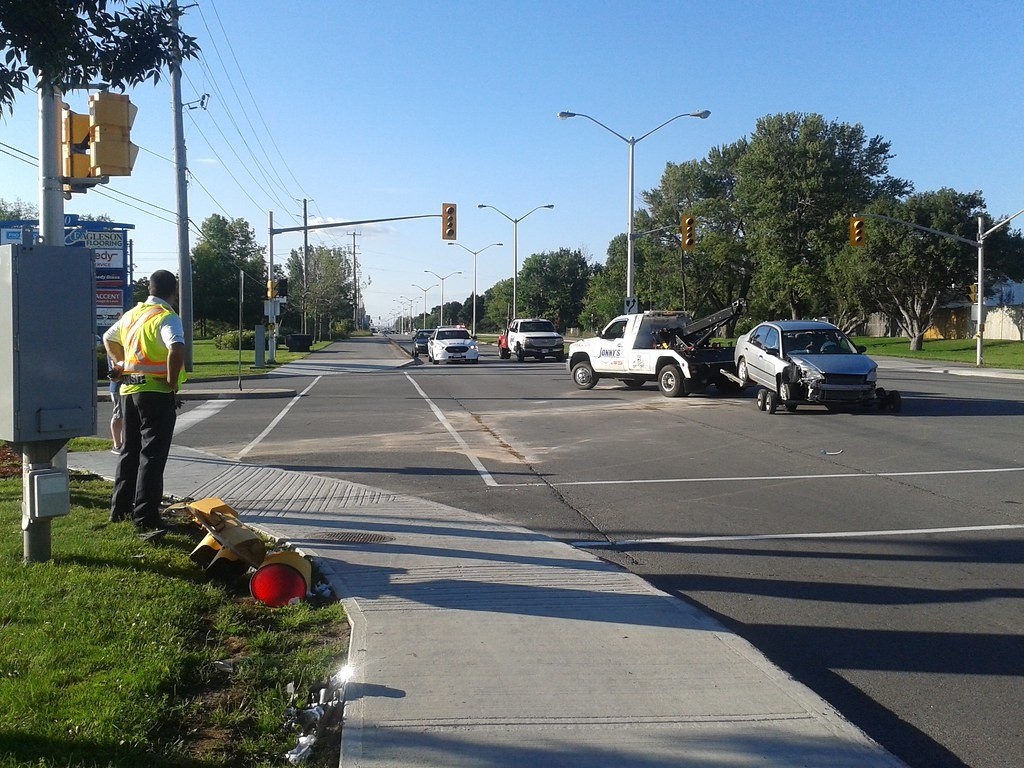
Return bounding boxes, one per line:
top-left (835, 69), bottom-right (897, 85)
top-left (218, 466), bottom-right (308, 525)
top-left (103, 270), bottom-right (187, 532)
top-left (106, 353), bottom-right (124, 456)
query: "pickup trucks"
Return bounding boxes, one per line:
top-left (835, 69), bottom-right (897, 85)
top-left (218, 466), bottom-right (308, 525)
top-left (566, 309), bottom-right (747, 399)
top-left (497, 319), bottom-right (565, 363)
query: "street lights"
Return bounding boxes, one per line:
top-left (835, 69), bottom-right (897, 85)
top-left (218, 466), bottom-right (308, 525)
top-left (447, 243), bottom-right (505, 337)
top-left (410, 283), bottom-right (440, 329)
top-left (477, 203), bottom-right (555, 320)
top-left (424, 271), bottom-right (463, 326)
top-left (556, 108), bottom-right (713, 297)
top-left (400, 296), bottom-right (422, 334)
top-left (388, 299), bottom-right (419, 334)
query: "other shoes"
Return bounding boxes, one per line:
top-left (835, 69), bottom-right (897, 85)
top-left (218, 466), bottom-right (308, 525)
top-left (111, 444), bottom-right (124, 455)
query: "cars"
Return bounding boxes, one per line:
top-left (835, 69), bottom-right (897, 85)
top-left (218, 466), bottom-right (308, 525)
top-left (427, 326), bottom-right (479, 365)
top-left (412, 329), bottom-right (436, 357)
top-left (370, 326), bottom-right (411, 336)
top-left (735, 320), bottom-right (877, 412)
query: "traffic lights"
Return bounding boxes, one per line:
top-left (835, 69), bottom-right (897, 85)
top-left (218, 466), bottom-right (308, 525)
top-left (59, 110), bottom-right (95, 193)
top-left (267, 280), bottom-right (279, 298)
top-left (966, 285), bottom-right (977, 303)
top-left (850, 216), bottom-right (866, 246)
top-left (682, 215), bottom-right (696, 250)
top-left (89, 91), bottom-right (141, 178)
top-left (186, 497), bottom-right (313, 609)
top-left (442, 203), bottom-right (456, 240)
